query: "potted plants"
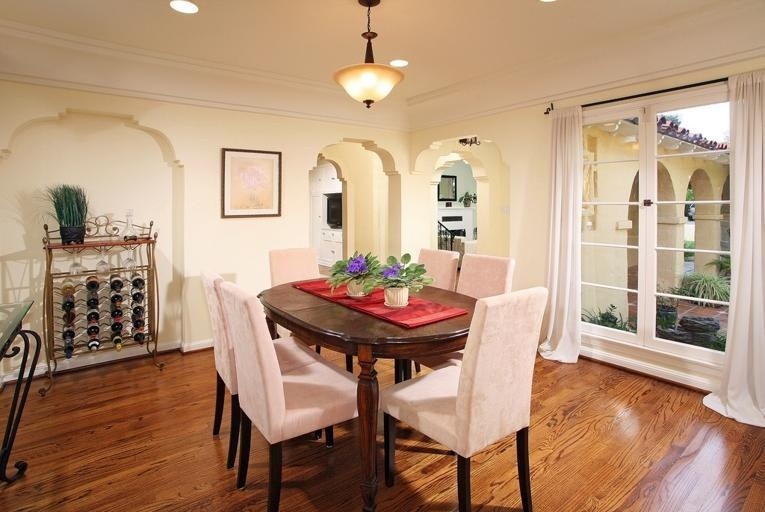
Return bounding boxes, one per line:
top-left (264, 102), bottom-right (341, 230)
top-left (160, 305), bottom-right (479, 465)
top-left (459, 192), bottom-right (477, 206)
top-left (656, 282), bottom-right (678, 329)
top-left (40, 183), bottom-right (87, 244)
top-left (686, 271), bottom-right (730, 308)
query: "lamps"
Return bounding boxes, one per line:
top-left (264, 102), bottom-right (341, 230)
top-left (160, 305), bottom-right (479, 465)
top-left (333, 1), bottom-right (405, 109)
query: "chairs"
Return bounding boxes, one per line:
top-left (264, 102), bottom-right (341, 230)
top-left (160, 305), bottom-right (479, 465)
top-left (194, 266), bottom-right (322, 468)
top-left (375, 286), bottom-right (549, 512)
top-left (268, 246), bottom-right (321, 353)
top-left (346, 246), bottom-right (460, 380)
top-left (219, 279), bottom-right (359, 512)
top-left (394, 253), bottom-right (516, 384)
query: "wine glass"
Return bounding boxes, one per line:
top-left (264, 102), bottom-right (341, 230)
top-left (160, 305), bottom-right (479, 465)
top-left (122, 248), bottom-right (136, 272)
top-left (95, 247), bottom-right (109, 276)
top-left (68, 250), bottom-right (88, 276)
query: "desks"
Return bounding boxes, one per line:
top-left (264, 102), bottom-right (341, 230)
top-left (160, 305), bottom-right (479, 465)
top-left (257, 277), bottom-right (478, 512)
top-left (0, 300), bottom-right (42, 481)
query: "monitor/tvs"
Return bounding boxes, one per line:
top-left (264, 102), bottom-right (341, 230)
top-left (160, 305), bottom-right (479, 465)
top-left (327, 196), bottom-right (342, 229)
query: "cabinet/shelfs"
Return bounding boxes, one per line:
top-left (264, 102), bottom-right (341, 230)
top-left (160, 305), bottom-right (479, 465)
top-left (310, 161), bottom-right (343, 268)
top-left (38, 215), bottom-right (165, 396)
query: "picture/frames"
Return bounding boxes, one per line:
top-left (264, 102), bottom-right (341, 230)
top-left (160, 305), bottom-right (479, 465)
top-left (437, 175), bottom-right (457, 201)
top-left (221, 148), bottom-right (281, 217)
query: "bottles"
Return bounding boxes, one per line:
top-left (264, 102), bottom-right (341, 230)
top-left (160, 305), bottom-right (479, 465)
top-left (122, 210), bottom-right (138, 241)
top-left (60, 271), bottom-right (146, 359)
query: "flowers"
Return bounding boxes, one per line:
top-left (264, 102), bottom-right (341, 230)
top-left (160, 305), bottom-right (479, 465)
top-left (326, 251), bottom-right (383, 295)
top-left (363, 253), bottom-right (433, 294)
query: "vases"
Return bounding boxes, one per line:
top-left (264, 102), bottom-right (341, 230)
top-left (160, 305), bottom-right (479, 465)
top-left (346, 280), bottom-right (367, 297)
top-left (382, 287), bottom-right (409, 307)
